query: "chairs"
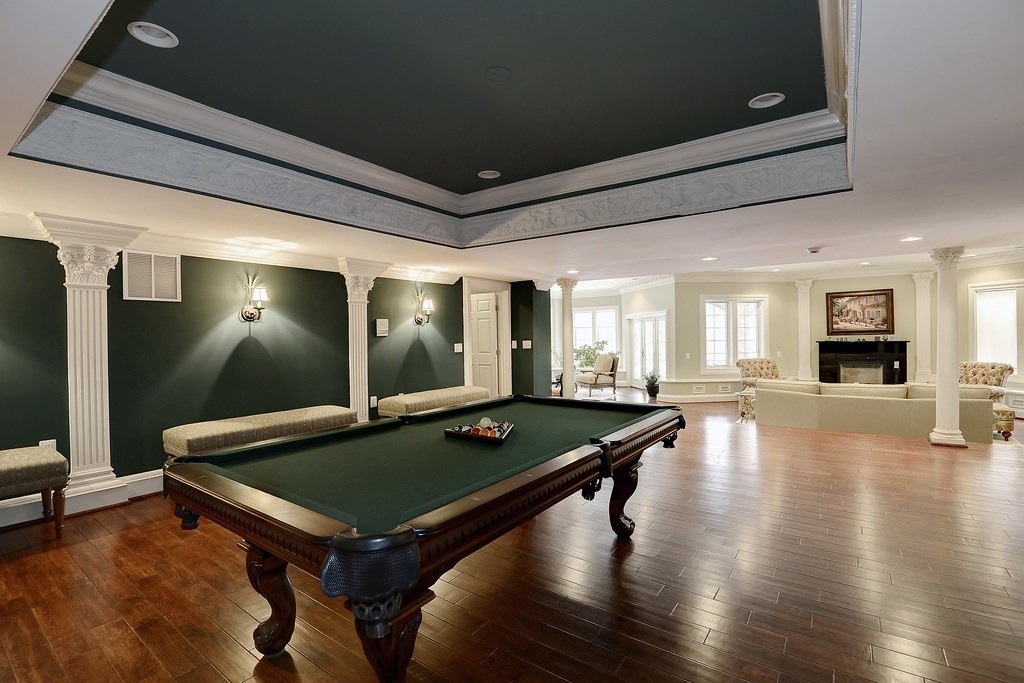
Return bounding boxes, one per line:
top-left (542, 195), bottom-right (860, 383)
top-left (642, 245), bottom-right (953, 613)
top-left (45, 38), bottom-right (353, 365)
top-left (552, 366), bottom-right (577, 397)
top-left (574, 353), bottom-right (619, 397)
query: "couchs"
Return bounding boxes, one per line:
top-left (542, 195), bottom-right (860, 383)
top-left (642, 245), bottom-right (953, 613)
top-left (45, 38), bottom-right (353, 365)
top-left (735, 358), bottom-right (1016, 442)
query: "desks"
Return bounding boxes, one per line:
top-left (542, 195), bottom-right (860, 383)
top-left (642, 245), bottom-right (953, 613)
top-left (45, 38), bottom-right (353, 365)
top-left (734, 392), bottom-right (755, 424)
top-left (164, 395), bottom-right (686, 683)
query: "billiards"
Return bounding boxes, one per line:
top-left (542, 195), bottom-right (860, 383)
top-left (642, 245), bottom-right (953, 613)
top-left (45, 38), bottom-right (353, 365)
top-left (451, 416), bottom-right (512, 438)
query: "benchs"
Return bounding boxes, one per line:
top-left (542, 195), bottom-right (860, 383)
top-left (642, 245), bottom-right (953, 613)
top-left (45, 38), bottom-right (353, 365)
top-left (162, 405), bottom-right (358, 459)
top-left (378, 386), bottom-right (491, 418)
top-left (0, 447), bottom-right (69, 539)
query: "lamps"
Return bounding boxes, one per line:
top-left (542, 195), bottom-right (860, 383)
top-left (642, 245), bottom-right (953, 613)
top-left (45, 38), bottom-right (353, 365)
top-left (415, 291), bottom-right (434, 326)
top-left (242, 275), bottom-right (269, 322)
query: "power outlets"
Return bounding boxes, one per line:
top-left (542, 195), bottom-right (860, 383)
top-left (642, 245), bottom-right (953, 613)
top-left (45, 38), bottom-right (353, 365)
top-left (370, 396), bottom-right (377, 408)
top-left (39, 439), bottom-right (56, 451)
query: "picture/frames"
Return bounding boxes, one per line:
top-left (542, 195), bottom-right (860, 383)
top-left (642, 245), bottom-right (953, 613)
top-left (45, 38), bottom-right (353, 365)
top-left (826, 289), bottom-right (894, 336)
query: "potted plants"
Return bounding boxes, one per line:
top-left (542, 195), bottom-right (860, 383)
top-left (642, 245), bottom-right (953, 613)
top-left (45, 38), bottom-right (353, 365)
top-left (643, 370), bottom-right (659, 397)
